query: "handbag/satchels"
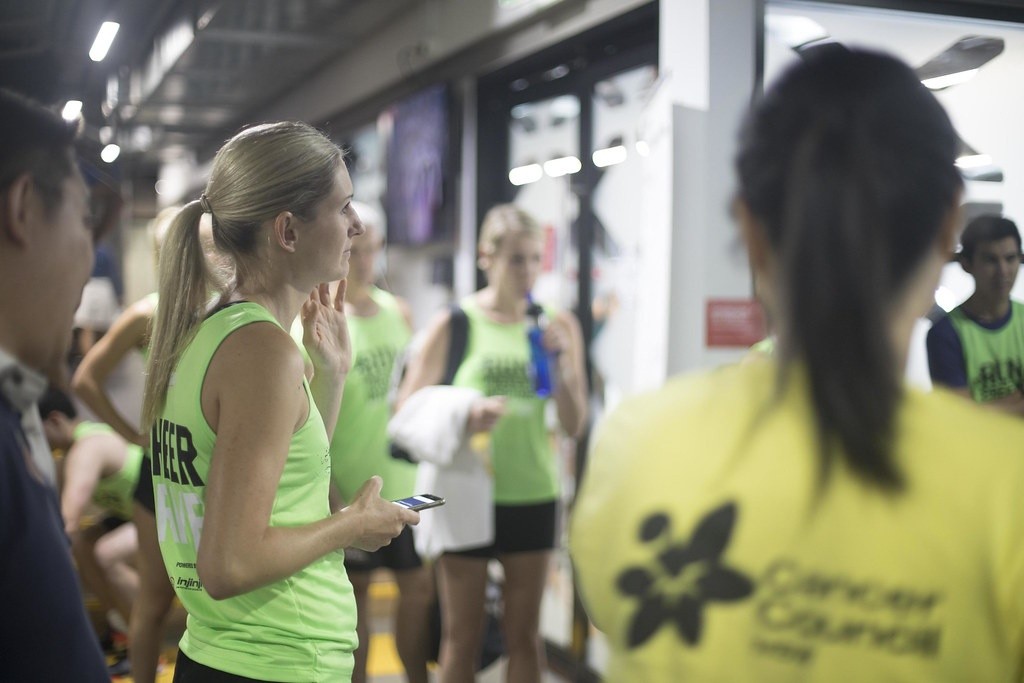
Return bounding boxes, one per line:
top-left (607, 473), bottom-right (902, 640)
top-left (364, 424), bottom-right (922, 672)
top-left (384, 302), bottom-right (470, 463)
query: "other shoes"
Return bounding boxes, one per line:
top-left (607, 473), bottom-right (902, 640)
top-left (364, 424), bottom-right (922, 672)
top-left (105, 648), bottom-right (130, 675)
top-left (96, 629), bottom-right (114, 648)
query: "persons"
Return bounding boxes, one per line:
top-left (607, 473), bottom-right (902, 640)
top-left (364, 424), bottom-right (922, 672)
top-left (0, 46), bottom-right (1024, 683)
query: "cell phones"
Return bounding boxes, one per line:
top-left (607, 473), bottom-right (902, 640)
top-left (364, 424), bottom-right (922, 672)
top-left (391, 494), bottom-right (446, 511)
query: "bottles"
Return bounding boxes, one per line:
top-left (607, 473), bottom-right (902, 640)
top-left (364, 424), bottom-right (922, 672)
top-left (521, 305), bottom-right (555, 395)
